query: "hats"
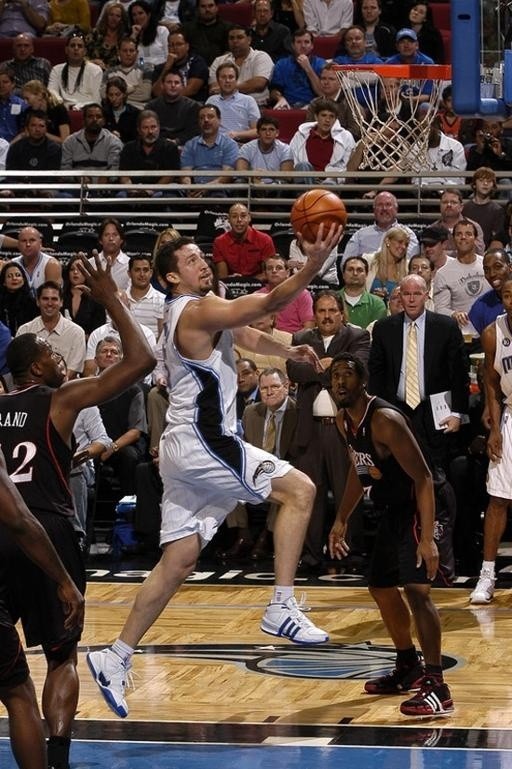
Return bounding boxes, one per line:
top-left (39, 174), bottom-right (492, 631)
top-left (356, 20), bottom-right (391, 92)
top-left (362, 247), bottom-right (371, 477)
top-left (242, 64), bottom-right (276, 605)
top-left (420, 227), bottom-right (448, 245)
top-left (396, 28), bottom-right (417, 41)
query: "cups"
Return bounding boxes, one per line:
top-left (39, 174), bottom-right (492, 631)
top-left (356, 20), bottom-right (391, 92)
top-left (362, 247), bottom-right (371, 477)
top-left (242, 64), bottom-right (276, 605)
top-left (462, 333), bottom-right (472, 343)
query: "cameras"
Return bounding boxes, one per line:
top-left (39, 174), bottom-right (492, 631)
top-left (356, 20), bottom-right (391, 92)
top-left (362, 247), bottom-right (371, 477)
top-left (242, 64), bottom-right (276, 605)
top-left (481, 128), bottom-right (496, 144)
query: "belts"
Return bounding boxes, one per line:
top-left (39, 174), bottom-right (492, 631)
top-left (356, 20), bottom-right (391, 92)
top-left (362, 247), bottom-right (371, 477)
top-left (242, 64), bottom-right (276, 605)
top-left (312, 416), bottom-right (336, 425)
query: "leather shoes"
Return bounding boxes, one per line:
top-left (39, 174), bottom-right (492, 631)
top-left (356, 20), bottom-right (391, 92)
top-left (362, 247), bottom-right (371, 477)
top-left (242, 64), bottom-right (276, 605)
top-left (248, 535), bottom-right (266, 560)
top-left (217, 537), bottom-right (251, 558)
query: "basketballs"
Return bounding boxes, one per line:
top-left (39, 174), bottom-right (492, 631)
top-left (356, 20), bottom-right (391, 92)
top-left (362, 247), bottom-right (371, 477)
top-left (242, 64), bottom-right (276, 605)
top-left (290, 189), bottom-right (347, 244)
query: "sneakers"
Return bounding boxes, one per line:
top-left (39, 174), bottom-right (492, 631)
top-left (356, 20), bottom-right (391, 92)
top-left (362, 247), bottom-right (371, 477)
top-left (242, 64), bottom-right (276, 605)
top-left (259, 592), bottom-right (329, 644)
top-left (86, 647), bottom-right (142, 718)
top-left (469, 568), bottom-right (499, 605)
top-left (364, 656), bottom-right (425, 694)
top-left (400, 674), bottom-right (455, 716)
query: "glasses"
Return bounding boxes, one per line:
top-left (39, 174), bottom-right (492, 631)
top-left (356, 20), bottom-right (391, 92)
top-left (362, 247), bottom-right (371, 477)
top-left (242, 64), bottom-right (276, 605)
top-left (259, 382), bottom-right (288, 393)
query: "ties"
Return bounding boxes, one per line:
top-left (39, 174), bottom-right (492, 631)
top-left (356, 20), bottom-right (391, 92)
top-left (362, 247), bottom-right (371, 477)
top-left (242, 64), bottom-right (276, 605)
top-left (405, 321), bottom-right (421, 411)
top-left (263, 413), bottom-right (276, 454)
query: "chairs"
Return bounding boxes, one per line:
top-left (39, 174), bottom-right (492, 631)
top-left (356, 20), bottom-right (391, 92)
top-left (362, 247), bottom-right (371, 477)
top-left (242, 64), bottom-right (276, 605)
top-left (261, 106), bottom-right (308, 146)
top-left (313, 32), bottom-right (345, 65)
top-left (438, 27), bottom-right (452, 65)
top-left (427, 3), bottom-right (452, 30)
top-left (38, 36), bottom-right (70, 66)
